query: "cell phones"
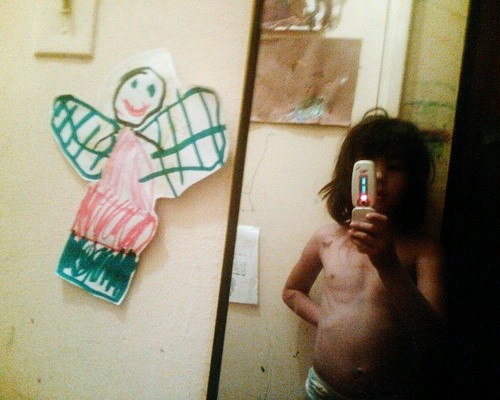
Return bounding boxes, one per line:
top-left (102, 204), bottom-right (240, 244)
top-left (350, 160), bottom-right (375, 231)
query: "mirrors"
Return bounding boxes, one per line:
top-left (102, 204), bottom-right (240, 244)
top-left (205, 0), bottom-right (469, 400)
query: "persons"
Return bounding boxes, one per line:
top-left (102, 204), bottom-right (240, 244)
top-left (283, 106), bottom-right (464, 400)
